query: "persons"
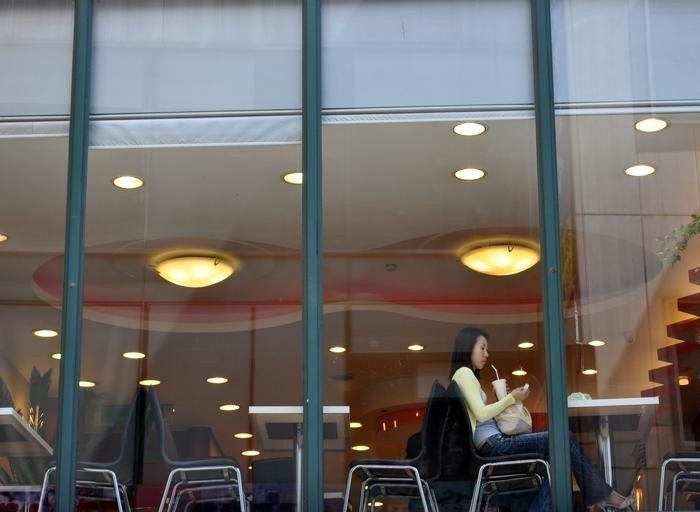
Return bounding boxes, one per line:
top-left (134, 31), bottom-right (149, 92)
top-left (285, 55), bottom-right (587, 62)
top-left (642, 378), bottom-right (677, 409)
top-left (449, 327), bottom-right (637, 511)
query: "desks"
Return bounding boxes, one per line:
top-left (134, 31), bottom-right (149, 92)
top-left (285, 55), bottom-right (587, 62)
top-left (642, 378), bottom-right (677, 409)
top-left (0, 407), bottom-right (54, 457)
top-left (567, 395), bottom-right (660, 511)
top-left (322, 491), bottom-right (344, 503)
top-left (0, 484), bottom-right (54, 512)
top-left (150, 389), bottom-right (244, 512)
top-left (249, 404), bottom-right (351, 511)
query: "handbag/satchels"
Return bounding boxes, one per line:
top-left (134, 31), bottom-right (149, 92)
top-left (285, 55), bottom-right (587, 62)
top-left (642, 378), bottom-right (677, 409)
top-left (494, 401), bottom-right (533, 435)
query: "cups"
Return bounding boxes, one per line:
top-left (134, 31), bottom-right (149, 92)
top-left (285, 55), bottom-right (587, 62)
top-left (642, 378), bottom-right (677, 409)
top-left (492, 377), bottom-right (507, 404)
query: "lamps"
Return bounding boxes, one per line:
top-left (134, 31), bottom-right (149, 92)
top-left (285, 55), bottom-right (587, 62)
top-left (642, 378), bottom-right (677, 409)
top-left (153, 251), bottom-right (238, 290)
top-left (457, 237), bottom-right (540, 277)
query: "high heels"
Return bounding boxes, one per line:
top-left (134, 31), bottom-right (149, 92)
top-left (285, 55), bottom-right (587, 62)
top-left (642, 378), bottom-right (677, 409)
top-left (597, 489), bottom-right (635, 512)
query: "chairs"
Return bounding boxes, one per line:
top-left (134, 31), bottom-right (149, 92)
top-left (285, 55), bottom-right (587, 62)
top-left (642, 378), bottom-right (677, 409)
top-left (37, 388), bottom-right (147, 512)
top-left (482, 473), bottom-right (542, 512)
top-left (75, 485), bottom-right (128, 512)
top-left (363, 407), bottom-right (451, 511)
top-left (172, 483), bottom-right (251, 512)
top-left (450, 380), bottom-right (552, 511)
top-left (658, 450), bottom-right (700, 512)
top-left (342, 379), bottom-right (448, 511)
top-left (664, 479), bottom-right (698, 512)
